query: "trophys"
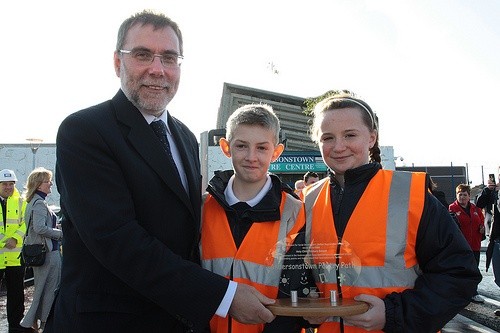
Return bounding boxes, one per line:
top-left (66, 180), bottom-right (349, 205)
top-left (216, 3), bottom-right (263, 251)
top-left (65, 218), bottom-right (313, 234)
top-left (263, 230), bottom-right (370, 317)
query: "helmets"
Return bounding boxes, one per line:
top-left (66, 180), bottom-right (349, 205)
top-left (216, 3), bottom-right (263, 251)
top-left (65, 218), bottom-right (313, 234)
top-left (0, 169), bottom-right (17, 181)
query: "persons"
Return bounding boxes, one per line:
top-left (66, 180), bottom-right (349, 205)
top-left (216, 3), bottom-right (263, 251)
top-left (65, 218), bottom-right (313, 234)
top-left (450, 165), bottom-right (500, 289)
top-left (198, 101), bottom-right (312, 333)
top-left (295, 170), bottom-right (319, 201)
top-left (18, 167), bottom-right (63, 333)
top-left (304, 95), bottom-right (483, 333)
top-left (0, 169), bottom-right (35, 332)
top-left (43, 8), bottom-right (276, 332)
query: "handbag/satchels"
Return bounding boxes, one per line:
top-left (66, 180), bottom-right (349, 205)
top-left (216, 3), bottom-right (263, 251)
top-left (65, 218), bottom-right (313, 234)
top-left (17, 243), bottom-right (46, 267)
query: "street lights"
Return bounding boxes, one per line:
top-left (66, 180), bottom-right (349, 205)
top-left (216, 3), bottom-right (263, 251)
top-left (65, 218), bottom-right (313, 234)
top-left (27, 138), bottom-right (43, 171)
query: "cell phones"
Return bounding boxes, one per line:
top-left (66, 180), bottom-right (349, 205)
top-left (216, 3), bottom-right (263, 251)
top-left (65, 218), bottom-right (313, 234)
top-left (489, 174), bottom-right (495, 184)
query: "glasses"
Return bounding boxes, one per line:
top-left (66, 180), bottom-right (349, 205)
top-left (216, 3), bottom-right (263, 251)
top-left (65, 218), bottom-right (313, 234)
top-left (118, 49), bottom-right (184, 68)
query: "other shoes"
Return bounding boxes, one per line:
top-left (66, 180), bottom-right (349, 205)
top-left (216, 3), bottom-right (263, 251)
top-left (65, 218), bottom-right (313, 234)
top-left (471, 295), bottom-right (484, 303)
top-left (8, 323), bottom-right (17, 333)
top-left (17, 324), bottom-right (39, 333)
